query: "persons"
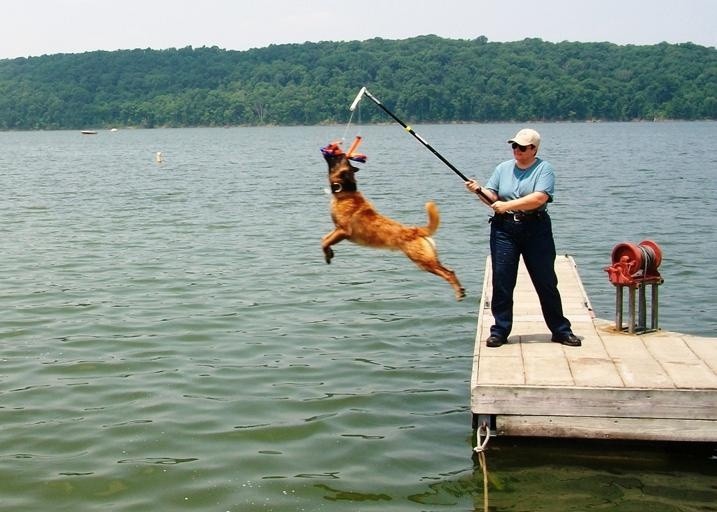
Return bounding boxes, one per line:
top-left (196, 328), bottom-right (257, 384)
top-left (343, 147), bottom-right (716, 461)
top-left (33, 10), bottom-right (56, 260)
top-left (464, 127), bottom-right (582, 348)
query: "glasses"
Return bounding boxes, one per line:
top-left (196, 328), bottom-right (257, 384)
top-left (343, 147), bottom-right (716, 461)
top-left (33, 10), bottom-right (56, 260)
top-left (512, 143), bottom-right (532, 152)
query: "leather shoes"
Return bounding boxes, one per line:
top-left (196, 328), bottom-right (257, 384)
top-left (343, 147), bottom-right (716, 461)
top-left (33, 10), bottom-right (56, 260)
top-left (487, 335), bottom-right (509, 347)
top-left (552, 331), bottom-right (581, 346)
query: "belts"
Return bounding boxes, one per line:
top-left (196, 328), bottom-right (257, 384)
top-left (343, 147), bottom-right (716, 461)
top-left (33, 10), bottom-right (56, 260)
top-left (495, 209), bottom-right (545, 222)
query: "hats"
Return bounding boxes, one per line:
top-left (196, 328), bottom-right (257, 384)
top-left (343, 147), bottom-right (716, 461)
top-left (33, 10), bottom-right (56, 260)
top-left (507, 128), bottom-right (541, 151)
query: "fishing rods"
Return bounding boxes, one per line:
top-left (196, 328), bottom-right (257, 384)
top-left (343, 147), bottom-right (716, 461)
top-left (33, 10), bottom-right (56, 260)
top-left (350, 86), bottom-right (495, 205)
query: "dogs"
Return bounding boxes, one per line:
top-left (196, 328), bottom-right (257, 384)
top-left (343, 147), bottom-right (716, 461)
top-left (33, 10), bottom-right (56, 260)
top-left (320, 153), bottom-right (467, 304)
